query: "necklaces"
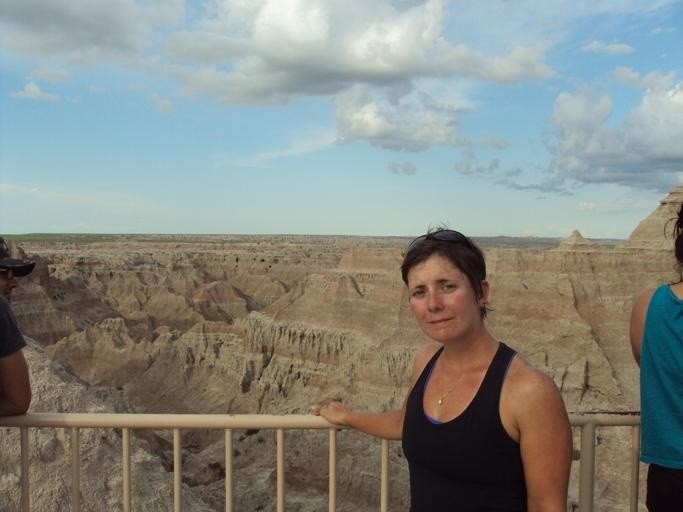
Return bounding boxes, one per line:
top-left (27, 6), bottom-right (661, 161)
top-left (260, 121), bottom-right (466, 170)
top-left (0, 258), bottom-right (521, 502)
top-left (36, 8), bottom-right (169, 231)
top-left (437, 349), bottom-right (487, 406)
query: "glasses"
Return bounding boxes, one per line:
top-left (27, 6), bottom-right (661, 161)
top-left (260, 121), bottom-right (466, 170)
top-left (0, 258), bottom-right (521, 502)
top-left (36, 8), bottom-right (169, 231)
top-left (406, 230), bottom-right (468, 257)
top-left (0, 265), bottom-right (16, 281)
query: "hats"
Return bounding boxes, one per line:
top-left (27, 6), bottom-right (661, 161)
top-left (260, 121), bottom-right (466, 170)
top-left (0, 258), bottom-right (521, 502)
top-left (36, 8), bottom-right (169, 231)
top-left (0, 237), bottom-right (36, 277)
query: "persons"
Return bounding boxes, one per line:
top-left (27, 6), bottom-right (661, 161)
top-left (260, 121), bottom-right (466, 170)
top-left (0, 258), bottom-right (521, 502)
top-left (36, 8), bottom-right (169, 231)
top-left (1, 234), bottom-right (36, 417)
top-left (627, 202), bottom-right (683, 512)
top-left (310, 219), bottom-right (573, 512)
